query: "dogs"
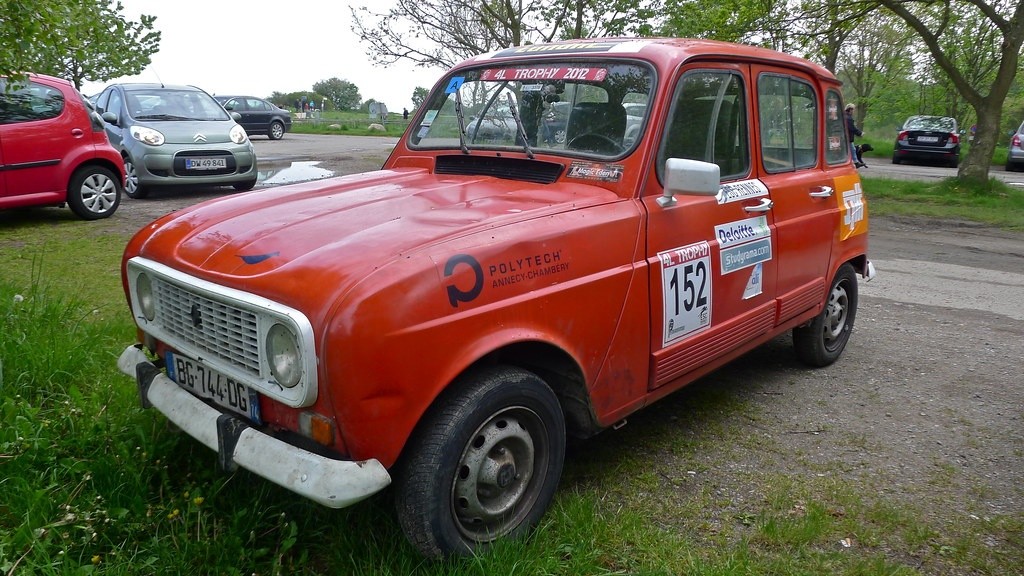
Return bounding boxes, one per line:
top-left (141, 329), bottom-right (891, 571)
top-left (855, 143), bottom-right (874, 168)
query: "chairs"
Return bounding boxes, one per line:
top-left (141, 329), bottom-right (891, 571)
top-left (565, 101), bottom-right (628, 153)
top-left (666, 99), bottom-right (734, 177)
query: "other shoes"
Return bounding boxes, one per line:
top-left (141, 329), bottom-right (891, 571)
top-left (854, 162), bottom-right (866, 168)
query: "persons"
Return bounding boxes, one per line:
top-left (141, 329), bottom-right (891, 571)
top-left (846, 103), bottom-right (865, 169)
top-left (310, 101), bottom-right (314, 111)
top-left (971, 124), bottom-right (976, 134)
top-left (383, 105), bottom-right (388, 120)
top-left (403, 108), bottom-right (408, 123)
top-left (1006, 128), bottom-right (1014, 149)
top-left (542, 102), bottom-right (556, 147)
top-left (294, 96), bottom-right (309, 112)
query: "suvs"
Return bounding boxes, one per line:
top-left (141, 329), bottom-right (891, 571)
top-left (542, 102), bottom-right (571, 143)
top-left (621, 102), bottom-right (646, 134)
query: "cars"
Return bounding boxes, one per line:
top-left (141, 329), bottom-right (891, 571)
top-left (467, 107), bottom-right (519, 140)
top-left (94, 84), bottom-right (258, 198)
top-left (892, 116), bottom-right (966, 168)
top-left (1006, 121), bottom-right (1024, 170)
top-left (189, 95), bottom-right (292, 141)
top-left (0, 71), bottom-right (125, 221)
top-left (87, 93), bottom-right (189, 114)
top-left (115, 37), bottom-right (875, 567)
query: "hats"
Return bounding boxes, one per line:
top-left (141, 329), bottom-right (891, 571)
top-left (845, 103), bottom-right (855, 110)
